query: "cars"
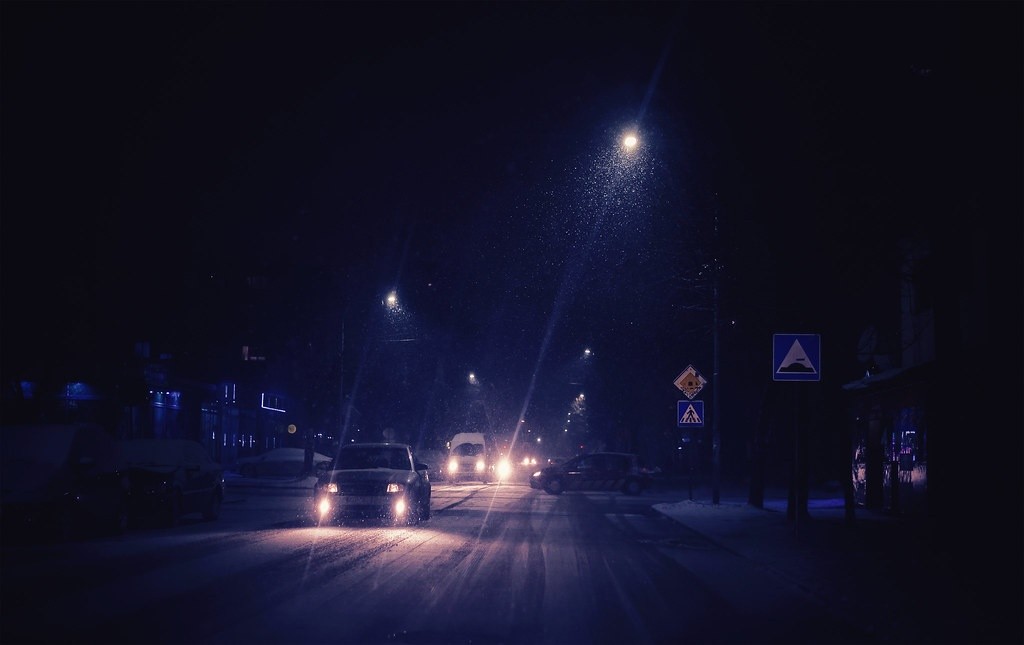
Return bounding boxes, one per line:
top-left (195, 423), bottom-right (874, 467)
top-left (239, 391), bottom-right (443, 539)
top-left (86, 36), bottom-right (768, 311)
top-left (529, 452), bottom-right (660, 497)
top-left (118, 439), bottom-right (225, 526)
top-left (447, 444), bottom-right (487, 481)
top-left (314, 442), bottom-right (430, 526)
